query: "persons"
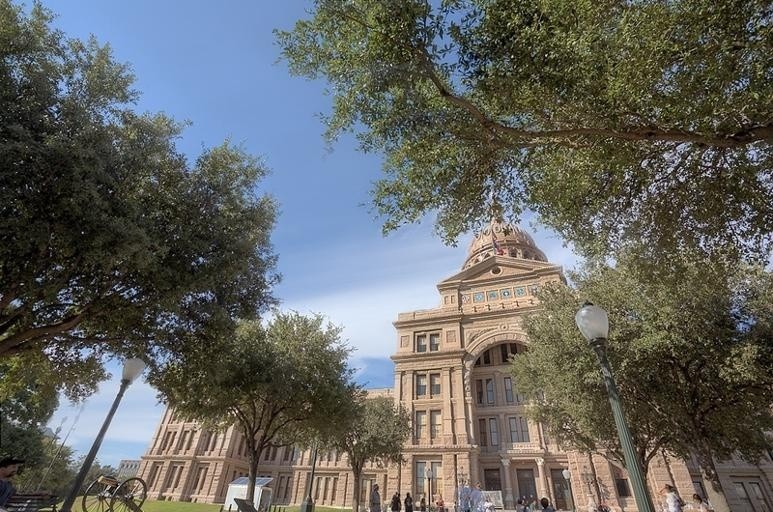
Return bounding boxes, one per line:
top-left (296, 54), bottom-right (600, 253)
top-left (692, 492), bottom-right (711, 511)
top-left (484, 494), bottom-right (496, 512)
top-left (473, 245), bottom-right (542, 265)
top-left (388, 490), bottom-right (399, 511)
top-left (396, 491), bottom-right (401, 511)
top-left (403, 491), bottom-right (413, 511)
top-left (515, 492), bottom-right (538, 512)
top-left (702, 495), bottom-right (714, 511)
top-left (0, 455), bottom-right (24, 512)
top-left (305, 493), bottom-right (312, 511)
top-left (540, 496), bottom-right (556, 511)
top-left (368, 483), bottom-right (381, 511)
top-left (419, 497), bottom-right (426, 511)
top-left (467, 481), bottom-right (485, 512)
top-left (458, 478), bottom-right (474, 511)
top-left (659, 481), bottom-right (685, 511)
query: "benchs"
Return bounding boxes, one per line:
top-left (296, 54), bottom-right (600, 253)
top-left (6, 490), bottom-right (60, 511)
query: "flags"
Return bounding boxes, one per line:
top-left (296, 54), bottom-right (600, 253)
top-left (492, 229), bottom-right (499, 252)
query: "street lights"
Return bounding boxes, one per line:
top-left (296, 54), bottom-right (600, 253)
top-left (425, 467), bottom-right (433, 512)
top-left (572, 295), bottom-right (655, 512)
top-left (56, 354), bottom-right (151, 512)
top-left (560, 468), bottom-right (576, 512)
